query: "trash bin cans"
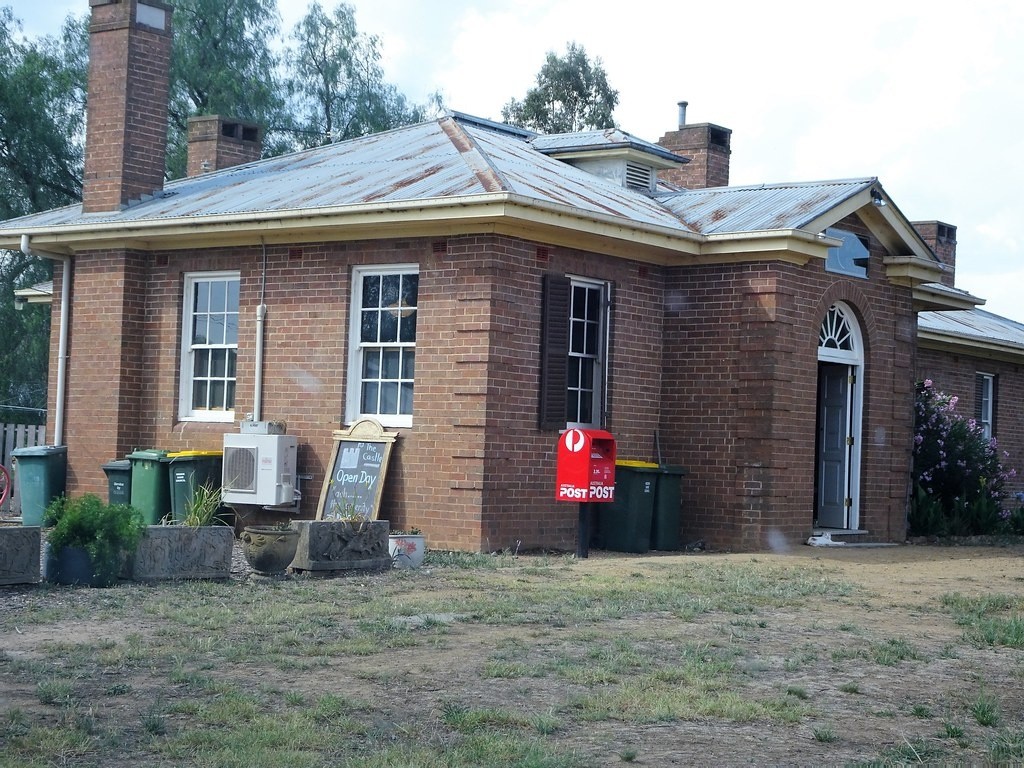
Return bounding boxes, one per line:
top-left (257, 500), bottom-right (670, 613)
top-left (125, 448), bottom-right (179, 526)
top-left (591, 460), bottom-right (659, 553)
top-left (10, 446), bottom-right (66, 528)
top-left (100, 460), bottom-right (131, 506)
top-left (651, 463), bottom-right (688, 552)
top-left (161, 451), bottom-right (224, 526)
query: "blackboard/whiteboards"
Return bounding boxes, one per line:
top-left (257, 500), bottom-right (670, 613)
top-left (313, 419), bottom-right (399, 522)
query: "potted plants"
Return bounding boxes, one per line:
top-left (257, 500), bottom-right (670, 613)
top-left (388, 527), bottom-right (424, 571)
top-left (239, 519), bottom-right (302, 582)
top-left (35, 490), bottom-right (146, 589)
top-left (289, 478), bottom-right (394, 569)
top-left (0, 526), bottom-right (42, 586)
top-left (134, 474), bottom-right (247, 582)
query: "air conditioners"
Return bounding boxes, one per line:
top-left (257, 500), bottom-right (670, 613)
top-left (220, 432), bottom-right (298, 505)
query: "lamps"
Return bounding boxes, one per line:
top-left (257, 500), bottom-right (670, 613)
top-left (14, 298), bottom-right (24, 309)
top-left (389, 297), bottom-right (414, 318)
top-left (870, 188), bottom-right (888, 207)
